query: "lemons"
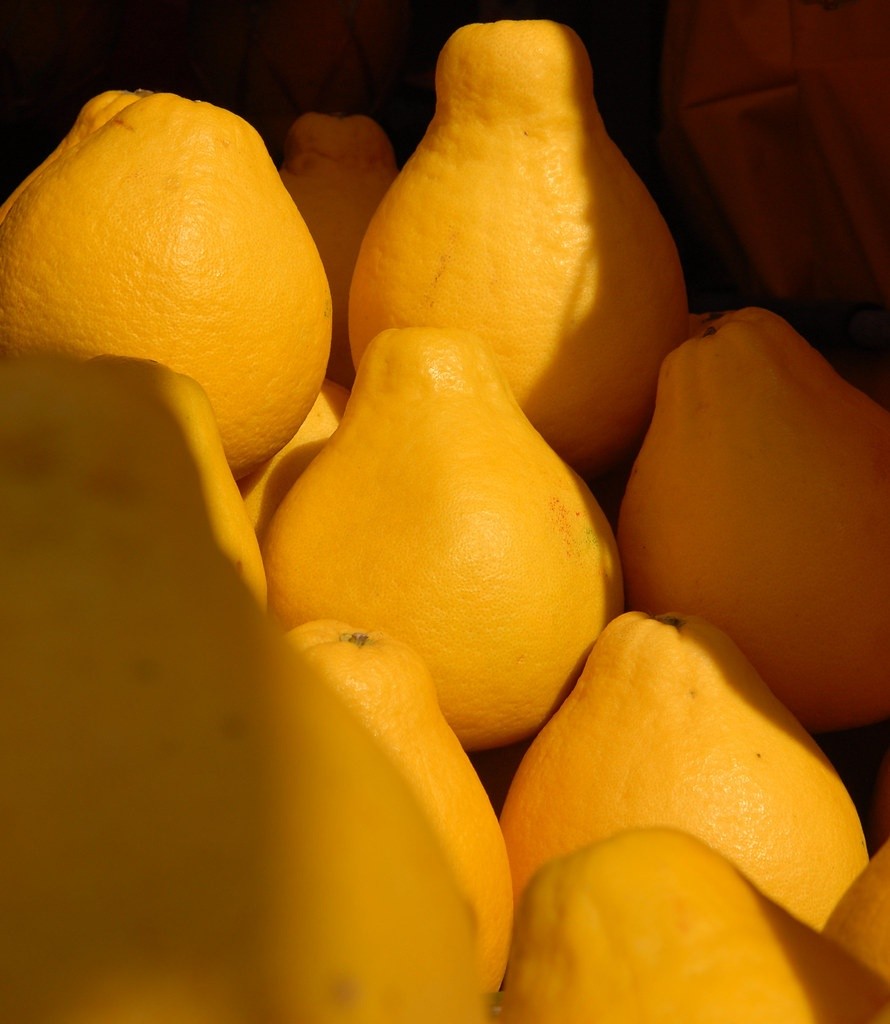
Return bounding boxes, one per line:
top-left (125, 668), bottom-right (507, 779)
top-left (0, 17), bottom-right (889, 1023)
